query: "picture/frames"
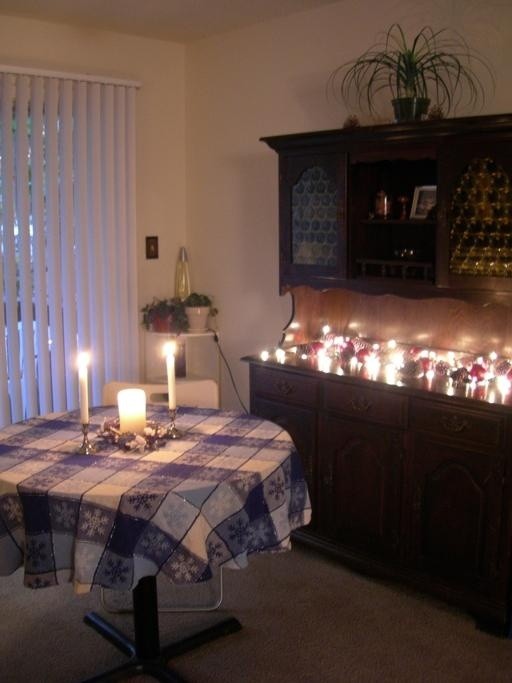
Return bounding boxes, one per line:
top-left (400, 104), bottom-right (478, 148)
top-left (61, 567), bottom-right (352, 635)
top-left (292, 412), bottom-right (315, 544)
top-left (408, 184), bottom-right (437, 220)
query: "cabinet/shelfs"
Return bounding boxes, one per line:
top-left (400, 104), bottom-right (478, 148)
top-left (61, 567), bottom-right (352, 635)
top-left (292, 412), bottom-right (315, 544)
top-left (237, 352), bottom-right (512, 639)
top-left (142, 332), bottom-right (221, 409)
top-left (258, 110), bottom-right (511, 313)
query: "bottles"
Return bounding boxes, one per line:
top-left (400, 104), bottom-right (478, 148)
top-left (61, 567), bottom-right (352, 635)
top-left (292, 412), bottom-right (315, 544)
top-left (373, 188), bottom-right (391, 217)
top-left (174, 246), bottom-right (190, 301)
top-left (397, 196), bottom-right (409, 221)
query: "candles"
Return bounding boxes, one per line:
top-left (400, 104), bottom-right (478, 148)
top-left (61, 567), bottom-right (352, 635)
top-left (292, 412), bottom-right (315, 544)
top-left (260, 323), bottom-right (511, 398)
top-left (163, 341), bottom-right (177, 409)
top-left (76, 351), bottom-right (90, 424)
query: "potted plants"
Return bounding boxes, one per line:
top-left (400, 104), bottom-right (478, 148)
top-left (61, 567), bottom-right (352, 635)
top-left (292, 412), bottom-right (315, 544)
top-left (322, 21), bottom-right (496, 122)
top-left (139, 295), bottom-right (190, 332)
top-left (182, 292), bottom-right (218, 331)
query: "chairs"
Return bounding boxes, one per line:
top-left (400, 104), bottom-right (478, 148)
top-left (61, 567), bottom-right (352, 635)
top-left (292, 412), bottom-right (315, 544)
top-left (102, 379), bottom-right (225, 612)
